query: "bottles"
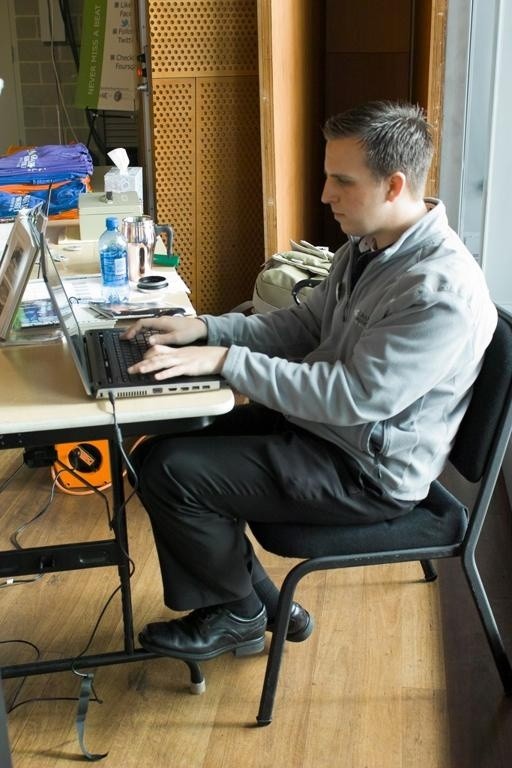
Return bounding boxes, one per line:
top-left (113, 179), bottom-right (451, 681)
top-left (97, 216), bottom-right (129, 287)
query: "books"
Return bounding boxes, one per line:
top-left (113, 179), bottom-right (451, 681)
top-left (89, 302), bottom-right (186, 320)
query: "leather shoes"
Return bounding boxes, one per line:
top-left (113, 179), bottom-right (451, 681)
top-left (266, 602), bottom-right (314, 643)
top-left (137, 603), bottom-right (268, 662)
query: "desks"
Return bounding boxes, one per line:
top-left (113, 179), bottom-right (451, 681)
top-left (0, 166), bottom-right (234, 695)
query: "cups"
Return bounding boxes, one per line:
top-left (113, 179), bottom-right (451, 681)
top-left (128, 243), bottom-right (147, 286)
top-left (122, 216), bottom-right (173, 274)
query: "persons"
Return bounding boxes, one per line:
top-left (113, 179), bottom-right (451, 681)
top-left (121, 98), bottom-right (497, 661)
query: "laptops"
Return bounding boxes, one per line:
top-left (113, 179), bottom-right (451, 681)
top-left (1, 211), bottom-right (39, 346)
top-left (37, 234), bottom-right (227, 399)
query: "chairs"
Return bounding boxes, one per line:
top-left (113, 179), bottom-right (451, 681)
top-left (237, 304), bottom-right (512, 727)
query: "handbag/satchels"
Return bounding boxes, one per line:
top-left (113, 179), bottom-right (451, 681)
top-left (250, 240), bottom-right (337, 313)
